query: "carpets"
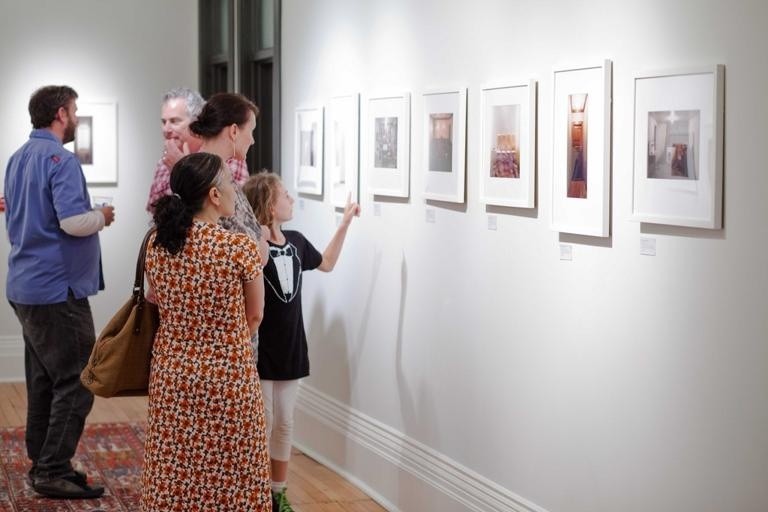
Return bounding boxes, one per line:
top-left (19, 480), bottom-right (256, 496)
top-left (0, 419), bottom-right (146, 512)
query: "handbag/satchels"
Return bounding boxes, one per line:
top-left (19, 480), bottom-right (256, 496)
top-left (79, 226), bottom-right (158, 399)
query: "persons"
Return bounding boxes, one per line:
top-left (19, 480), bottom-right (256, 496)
top-left (2, 86), bottom-right (115, 499)
top-left (140, 154), bottom-right (273, 512)
top-left (146, 86), bottom-right (261, 243)
top-left (189, 92), bottom-right (270, 364)
top-left (242, 170), bottom-right (360, 512)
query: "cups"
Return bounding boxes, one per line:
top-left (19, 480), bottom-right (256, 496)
top-left (91, 195), bottom-right (115, 209)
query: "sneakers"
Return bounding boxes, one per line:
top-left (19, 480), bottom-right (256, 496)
top-left (272, 489), bottom-right (295, 512)
top-left (26, 471), bottom-right (104, 497)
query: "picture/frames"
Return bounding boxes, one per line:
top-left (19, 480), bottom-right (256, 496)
top-left (63, 100), bottom-right (119, 185)
top-left (325, 93), bottom-right (360, 209)
top-left (362, 90), bottom-right (412, 199)
top-left (418, 85), bottom-right (467, 205)
top-left (478, 78), bottom-right (537, 211)
top-left (631, 62), bottom-right (722, 230)
top-left (295, 106), bottom-right (323, 198)
top-left (549, 58), bottom-right (609, 240)
